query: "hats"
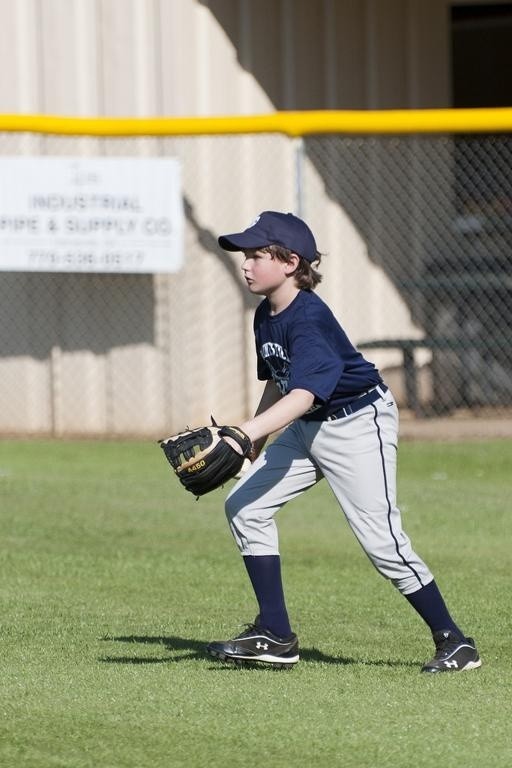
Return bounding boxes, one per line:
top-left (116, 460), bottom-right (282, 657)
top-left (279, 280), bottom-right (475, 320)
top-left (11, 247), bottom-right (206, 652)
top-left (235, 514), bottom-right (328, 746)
top-left (218, 210), bottom-right (317, 262)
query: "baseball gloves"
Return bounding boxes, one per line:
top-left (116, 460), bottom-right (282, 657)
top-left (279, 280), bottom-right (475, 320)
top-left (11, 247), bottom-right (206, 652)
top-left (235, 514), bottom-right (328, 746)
top-left (159, 426), bottom-right (252, 497)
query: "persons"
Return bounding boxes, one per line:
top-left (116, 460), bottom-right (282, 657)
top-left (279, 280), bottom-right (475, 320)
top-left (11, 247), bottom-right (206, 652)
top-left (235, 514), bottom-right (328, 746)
top-left (156, 210), bottom-right (485, 677)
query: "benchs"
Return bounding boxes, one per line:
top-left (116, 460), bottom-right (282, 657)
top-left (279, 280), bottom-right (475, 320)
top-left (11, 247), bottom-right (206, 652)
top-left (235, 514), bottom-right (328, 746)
top-left (352, 205), bottom-right (512, 416)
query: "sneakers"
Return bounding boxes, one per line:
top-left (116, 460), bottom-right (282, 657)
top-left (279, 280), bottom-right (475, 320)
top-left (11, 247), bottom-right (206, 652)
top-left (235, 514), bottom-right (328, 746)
top-left (208, 623), bottom-right (299, 668)
top-left (422, 632), bottom-right (482, 675)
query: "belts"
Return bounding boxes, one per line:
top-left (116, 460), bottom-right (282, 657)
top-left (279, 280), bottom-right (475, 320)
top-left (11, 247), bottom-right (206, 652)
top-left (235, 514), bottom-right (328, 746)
top-left (299, 382), bottom-right (389, 421)
